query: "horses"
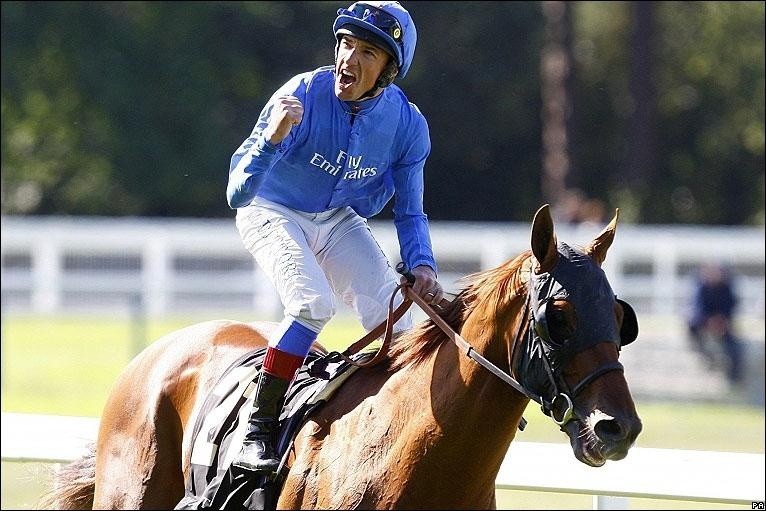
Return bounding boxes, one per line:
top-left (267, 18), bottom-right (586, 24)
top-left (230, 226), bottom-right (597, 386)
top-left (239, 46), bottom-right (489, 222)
top-left (46, 203), bottom-right (645, 510)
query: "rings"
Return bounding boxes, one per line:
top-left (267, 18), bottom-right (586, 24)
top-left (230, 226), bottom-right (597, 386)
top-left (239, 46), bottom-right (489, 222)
top-left (427, 292), bottom-right (435, 297)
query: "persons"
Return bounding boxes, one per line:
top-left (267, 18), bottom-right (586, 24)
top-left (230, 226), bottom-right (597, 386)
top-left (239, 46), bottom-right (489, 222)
top-left (225, 1), bottom-right (445, 478)
top-left (682, 262), bottom-right (746, 384)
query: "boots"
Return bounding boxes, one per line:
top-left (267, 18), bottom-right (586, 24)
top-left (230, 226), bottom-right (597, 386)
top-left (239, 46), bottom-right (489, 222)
top-left (232, 368), bottom-right (290, 472)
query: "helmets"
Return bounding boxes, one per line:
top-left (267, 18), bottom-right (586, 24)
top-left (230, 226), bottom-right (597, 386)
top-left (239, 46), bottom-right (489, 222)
top-left (333, 0), bottom-right (419, 88)
